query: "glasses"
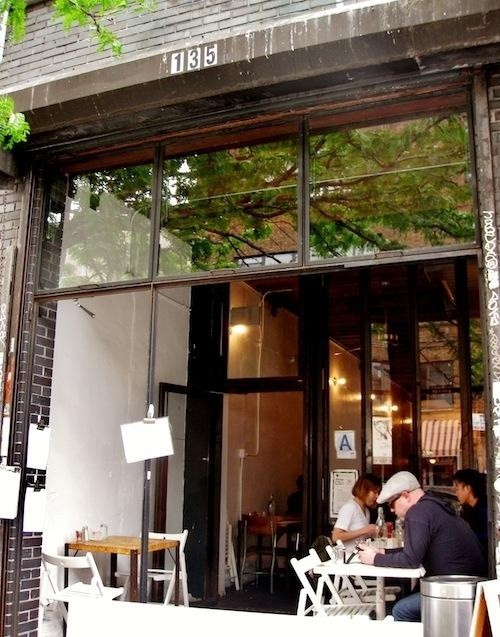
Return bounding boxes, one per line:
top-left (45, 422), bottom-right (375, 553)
top-left (388, 495), bottom-right (401, 508)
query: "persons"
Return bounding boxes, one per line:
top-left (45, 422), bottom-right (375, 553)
top-left (276, 474), bottom-right (302, 576)
top-left (358, 471), bottom-right (484, 621)
top-left (453, 469), bottom-right (490, 578)
top-left (331, 473), bottom-right (381, 564)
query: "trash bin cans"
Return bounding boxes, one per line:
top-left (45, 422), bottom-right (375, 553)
top-left (417, 572), bottom-right (490, 637)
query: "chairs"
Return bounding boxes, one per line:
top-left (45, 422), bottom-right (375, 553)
top-left (237, 512), bottom-right (289, 594)
top-left (38, 527), bottom-right (189, 625)
top-left (289, 534), bottom-right (402, 618)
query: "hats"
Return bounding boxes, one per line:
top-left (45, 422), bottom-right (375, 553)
top-left (377, 471), bottom-right (420, 504)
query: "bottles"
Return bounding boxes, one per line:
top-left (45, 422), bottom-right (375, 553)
top-left (392, 520), bottom-right (404, 549)
top-left (374, 507), bottom-right (388, 549)
top-left (76, 524), bottom-right (110, 544)
top-left (267, 493), bottom-right (276, 516)
